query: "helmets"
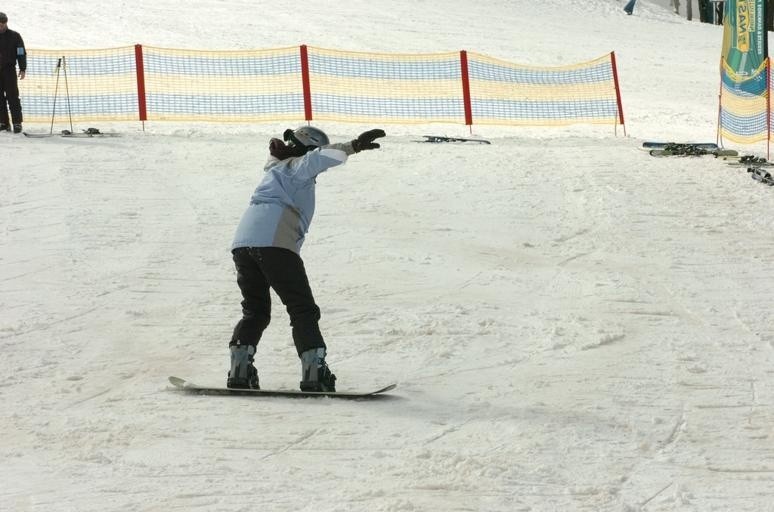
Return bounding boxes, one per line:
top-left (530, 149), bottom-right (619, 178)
top-left (289, 126), bottom-right (329, 153)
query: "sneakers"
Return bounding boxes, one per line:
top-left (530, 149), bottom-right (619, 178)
top-left (13, 122), bottom-right (22, 133)
top-left (0, 121), bottom-right (10, 132)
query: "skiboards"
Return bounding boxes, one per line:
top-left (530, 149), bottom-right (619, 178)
top-left (747, 169), bottom-right (774, 186)
top-left (727, 158), bottom-right (770, 167)
top-left (643, 142), bottom-right (733, 155)
top-left (24, 132), bottom-right (125, 139)
top-left (412, 135), bottom-right (490, 143)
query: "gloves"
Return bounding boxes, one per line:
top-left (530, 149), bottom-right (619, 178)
top-left (352, 129), bottom-right (386, 153)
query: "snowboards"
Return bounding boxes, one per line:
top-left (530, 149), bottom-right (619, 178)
top-left (169, 376), bottom-right (396, 396)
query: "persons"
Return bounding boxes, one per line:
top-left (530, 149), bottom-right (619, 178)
top-left (226, 126), bottom-right (386, 392)
top-left (0, 12), bottom-right (26, 133)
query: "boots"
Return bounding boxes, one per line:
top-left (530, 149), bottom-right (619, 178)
top-left (298, 347), bottom-right (337, 392)
top-left (227, 343), bottom-right (260, 389)
